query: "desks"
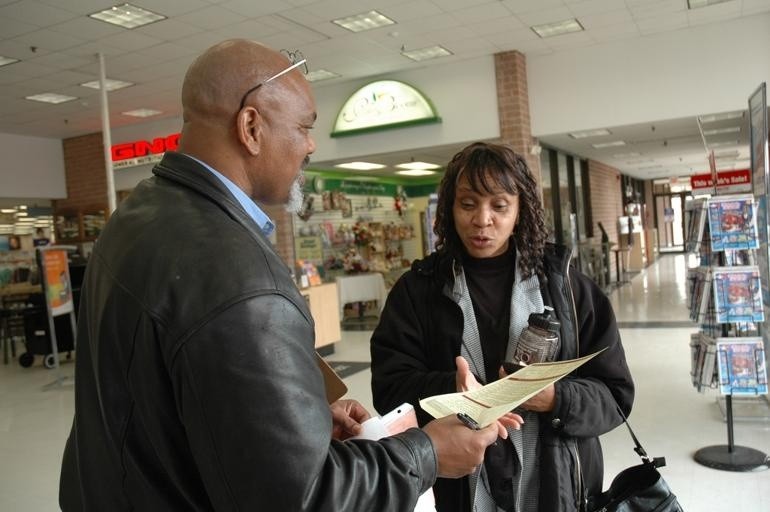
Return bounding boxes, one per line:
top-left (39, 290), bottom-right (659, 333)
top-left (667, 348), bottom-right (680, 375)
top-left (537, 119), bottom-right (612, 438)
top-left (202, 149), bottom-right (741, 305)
top-left (336, 272), bottom-right (383, 323)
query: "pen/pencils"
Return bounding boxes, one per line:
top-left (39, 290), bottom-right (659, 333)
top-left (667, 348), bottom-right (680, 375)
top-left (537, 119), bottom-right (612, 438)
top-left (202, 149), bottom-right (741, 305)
top-left (457, 413), bottom-right (499, 448)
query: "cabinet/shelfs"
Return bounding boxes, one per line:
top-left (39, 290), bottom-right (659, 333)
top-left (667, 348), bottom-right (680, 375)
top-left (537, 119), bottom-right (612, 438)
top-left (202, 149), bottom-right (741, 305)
top-left (684, 186), bottom-right (770, 470)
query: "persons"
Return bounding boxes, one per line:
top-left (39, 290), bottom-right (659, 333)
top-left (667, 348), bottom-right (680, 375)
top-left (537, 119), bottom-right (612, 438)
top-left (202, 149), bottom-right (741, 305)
top-left (33, 228), bottom-right (49, 245)
top-left (59, 271), bottom-right (68, 299)
top-left (10, 238), bottom-right (20, 250)
top-left (369, 141), bottom-right (635, 512)
top-left (58, 39), bottom-right (498, 511)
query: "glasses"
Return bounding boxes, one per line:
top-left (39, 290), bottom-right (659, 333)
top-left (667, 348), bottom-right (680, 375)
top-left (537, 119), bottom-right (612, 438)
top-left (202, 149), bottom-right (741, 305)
top-left (238, 46), bottom-right (310, 112)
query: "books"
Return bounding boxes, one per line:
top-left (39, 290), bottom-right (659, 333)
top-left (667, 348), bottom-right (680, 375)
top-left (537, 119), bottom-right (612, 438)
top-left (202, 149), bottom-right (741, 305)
top-left (684, 192), bottom-right (768, 396)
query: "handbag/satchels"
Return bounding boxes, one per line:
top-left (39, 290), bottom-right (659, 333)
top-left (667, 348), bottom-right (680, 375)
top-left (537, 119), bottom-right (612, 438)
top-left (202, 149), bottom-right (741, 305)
top-left (575, 376), bottom-right (687, 512)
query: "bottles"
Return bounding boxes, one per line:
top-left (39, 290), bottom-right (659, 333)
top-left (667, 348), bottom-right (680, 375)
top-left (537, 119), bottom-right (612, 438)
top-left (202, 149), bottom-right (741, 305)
top-left (510, 305), bottom-right (562, 367)
